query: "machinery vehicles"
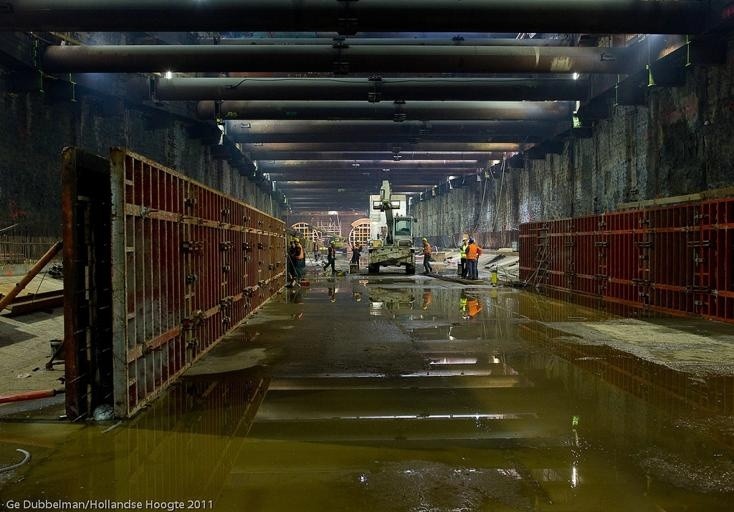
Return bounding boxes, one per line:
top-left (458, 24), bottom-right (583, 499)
top-left (368, 180), bottom-right (416, 275)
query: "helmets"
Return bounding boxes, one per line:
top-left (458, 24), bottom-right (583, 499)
top-left (463, 238), bottom-right (468, 242)
top-left (330, 241), bottom-right (335, 244)
top-left (294, 238), bottom-right (299, 242)
top-left (422, 238), bottom-right (427, 241)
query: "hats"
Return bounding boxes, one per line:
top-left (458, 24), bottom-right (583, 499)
top-left (469, 239), bottom-right (474, 243)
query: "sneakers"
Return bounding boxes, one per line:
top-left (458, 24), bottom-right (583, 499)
top-left (424, 268), bottom-right (432, 274)
top-left (332, 270), bottom-right (337, 272)
top-left (460, 276), bottom-right (478, 280)
top-left (323, 267), bottom-right (326, 271)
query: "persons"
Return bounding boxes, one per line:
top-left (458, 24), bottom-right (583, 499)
top-left (459, 237), bottom-right (469, 276)
top-left (419, 237), bottom-right (433, 273)
top-left (459, 288), bottom-right (483, 321)
top-left (350, 276), bottom-right (363, 303)
top-left (290, 237), bottom-right (306, 279)
top-left (311, 242), bottom-right (320, 260)
top-left (322, 241), bottom-right (338, 273)
top-left (351, 242), bottom-right (363, 270)
top-left (322, 272), bottom-right (342, 303)
top-left (461, 240), bottom-right (479, 279)
top-left (421, 278), bottom-right (433, 311)
top-left (465, 238), bottom-right (481, 279)
